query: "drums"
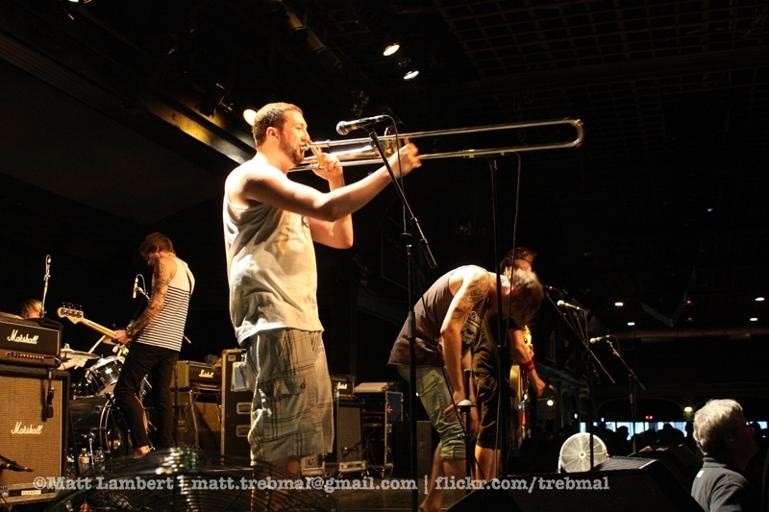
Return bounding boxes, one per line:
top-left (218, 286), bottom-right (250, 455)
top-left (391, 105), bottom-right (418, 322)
top-left (72, 393), bottom-right (147, 459)
top-left (85, 357), bottom-right (123, 397)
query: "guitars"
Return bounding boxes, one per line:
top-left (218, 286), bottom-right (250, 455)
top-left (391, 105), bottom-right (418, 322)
top-left (57, 302), bottom-right (118, 338)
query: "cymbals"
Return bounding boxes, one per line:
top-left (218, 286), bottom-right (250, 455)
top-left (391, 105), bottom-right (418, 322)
top-left (60, 343), bottom-right (100, 359)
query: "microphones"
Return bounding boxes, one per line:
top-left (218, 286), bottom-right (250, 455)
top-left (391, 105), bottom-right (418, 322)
top-left (557, 298), bottom-right (582, 312)
top-left (132, 277), bottom-right (139, 299)
top-left (588, 334), bottom-right (611, 344)
top-left (335, 114), bottom-right (388, 136)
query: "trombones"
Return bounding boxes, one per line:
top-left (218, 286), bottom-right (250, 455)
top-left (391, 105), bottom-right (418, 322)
top-left (288, 117), bottom-right (584, 173)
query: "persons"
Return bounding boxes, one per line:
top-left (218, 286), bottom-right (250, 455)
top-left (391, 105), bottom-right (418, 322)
top-left (389, 264), bottom-right (547, 512)
top-left (225, 101), bottom-right (421, 512)
top-left (616, 426), bottom-right (630, 440)
top-left (21, 300), bottom-right (45, 319)
top-left (683, 408), bottom-right (703, 444)
top-left (498, 249), bottom-right (546, 444)
top-left (111, 233), bottom-right (196, 465)
top-left (692, 398), bottom-right (758, 512)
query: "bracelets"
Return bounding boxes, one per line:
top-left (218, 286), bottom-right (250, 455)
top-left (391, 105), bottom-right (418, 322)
top-left (523, 360), bottom-right (535, 374)
